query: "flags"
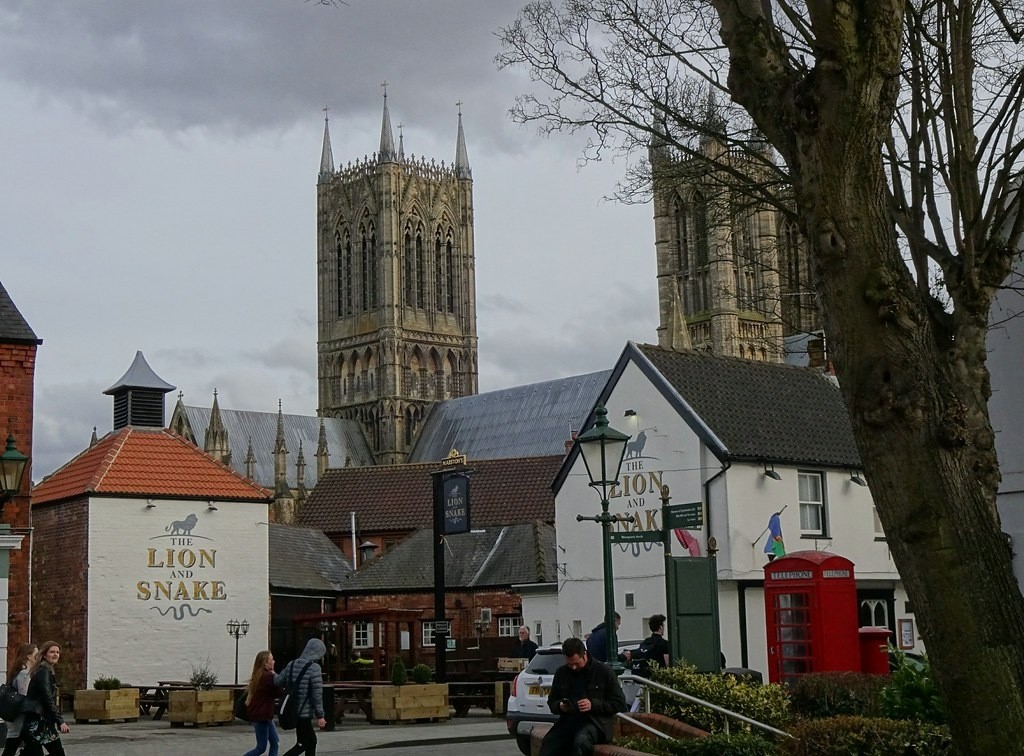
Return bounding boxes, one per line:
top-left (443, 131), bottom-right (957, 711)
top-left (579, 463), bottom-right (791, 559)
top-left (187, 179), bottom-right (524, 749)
top-left (763, 513), bottom-right (785, 557)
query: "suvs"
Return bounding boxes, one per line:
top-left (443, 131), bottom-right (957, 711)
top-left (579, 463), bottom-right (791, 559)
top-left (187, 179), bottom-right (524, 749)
top-left (505, 638), bottom-right (644, 756)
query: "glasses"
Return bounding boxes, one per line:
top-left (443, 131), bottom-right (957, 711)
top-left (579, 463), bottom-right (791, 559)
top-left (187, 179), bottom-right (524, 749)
top-left (520, 631), bottom-right (524, 634)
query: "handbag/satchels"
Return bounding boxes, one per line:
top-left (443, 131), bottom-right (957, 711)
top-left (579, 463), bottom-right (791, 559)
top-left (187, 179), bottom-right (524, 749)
top-left (0, 682), bottom-right (25, 723)
top-left (232, 691), bottom-right (254, 721)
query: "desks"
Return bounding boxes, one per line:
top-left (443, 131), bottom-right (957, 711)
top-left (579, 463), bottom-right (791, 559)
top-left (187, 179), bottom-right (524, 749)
top-left (121, 685), bottom-right (194, 721)
top-left (324, 682), bottom-right (375, 724)
top-left (444, 682), bottom-right (496, 717)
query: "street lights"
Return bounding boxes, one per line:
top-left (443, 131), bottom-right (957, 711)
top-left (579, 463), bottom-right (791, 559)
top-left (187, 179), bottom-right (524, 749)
top-left (226, 617), bottom-right (250, 684)
top-left (573, 401), bottom-right (633, 678)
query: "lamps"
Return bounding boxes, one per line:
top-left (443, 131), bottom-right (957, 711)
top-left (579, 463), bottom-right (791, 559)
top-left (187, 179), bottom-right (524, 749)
top-left (624, 410), bottom-right (636, 417)
top-left (146, 499), bottom-right (157, 508)
top-left (0, 429), bottom-right (33, 517)
top-left (849, 470), bottom-right (867, 488)
top-left (764, 464), bottom-right (782, 479)
top-left (210, 501), bottom-right (217, 511)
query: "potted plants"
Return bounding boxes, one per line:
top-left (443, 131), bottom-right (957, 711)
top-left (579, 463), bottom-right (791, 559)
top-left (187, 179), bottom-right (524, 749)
top-left (167, 663), bottom-right (235, 724)
top-left (373, 658), bottom-right (450, 721)
top-left (75, 676), bottom-right (139, 725)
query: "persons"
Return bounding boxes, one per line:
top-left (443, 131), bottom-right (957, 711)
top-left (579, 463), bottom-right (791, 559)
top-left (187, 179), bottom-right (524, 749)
top-left (243, 651), bottom-right (283, 756)
top-left (17, 641), bottom-right (70, 756)
top-left (380, 649), bottom-right (386, 664)
top-left (2, 643), bottom-right (44, 756)
top-left (350, 651), bottom-right (374, 664)
top-left (510, 625), bottom-right (538, 663)
top-left (539, 638), bottom-right (628, 756)
top-left (639, 614), bottom-right (669, 669)
top-left (586, 612), bottom-right (631, 662)
top-left (274, 638), bottom-right (327, 756)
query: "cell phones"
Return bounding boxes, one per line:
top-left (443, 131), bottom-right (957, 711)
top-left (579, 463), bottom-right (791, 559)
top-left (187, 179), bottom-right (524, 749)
top-left (562, 699), bottom-right (574, 710)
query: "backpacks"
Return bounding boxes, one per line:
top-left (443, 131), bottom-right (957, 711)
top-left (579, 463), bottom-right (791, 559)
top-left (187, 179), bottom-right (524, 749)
top-left (278, 659), bottom-right (315, 730)
top-left (631, 643), bottom-right (652, 685)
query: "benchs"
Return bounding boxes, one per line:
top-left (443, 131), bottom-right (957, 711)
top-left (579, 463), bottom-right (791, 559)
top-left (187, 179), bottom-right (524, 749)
top-left (336, 697), bottom-right (371, 704)
top-left (447, 694), bottom-right (495, 700)
top-left (138, 699), bottom-right (168, 707)
